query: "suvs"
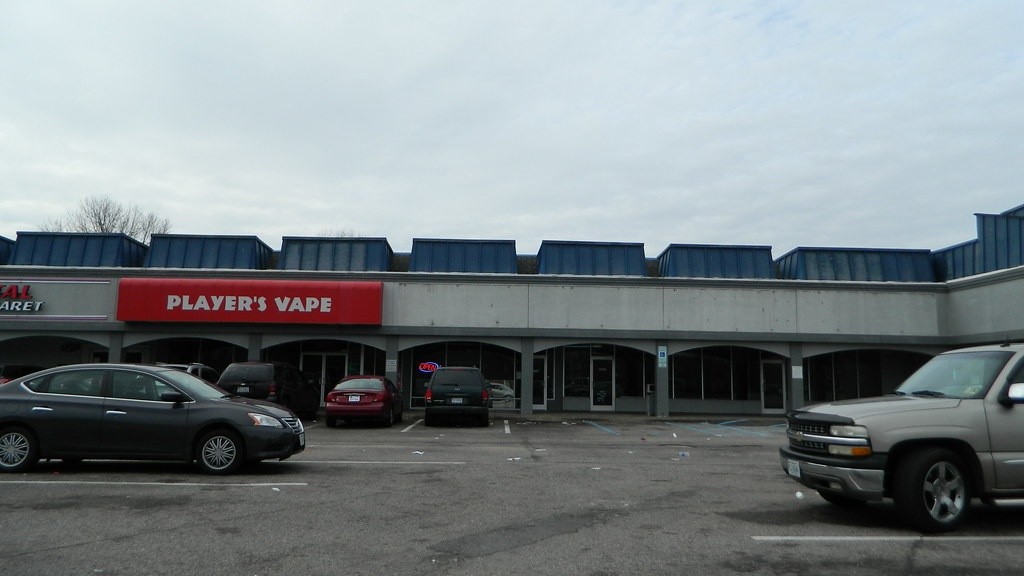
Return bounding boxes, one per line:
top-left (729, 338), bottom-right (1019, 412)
top-left (133, 360), bottom-right (221, 396)
top-left (780, 341), bottom-right (1023, 535)
top-left (214, 359), bottom-right (320, 422)
top-left (423, 364), bottom-right (491, 428)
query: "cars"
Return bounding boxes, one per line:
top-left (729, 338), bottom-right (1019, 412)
top-left (324, 375), bottom-right (405, 428)
top-left (0, 362), bottom-right (307, 476)
top-left (483, 380), bottom-right (515, 403)
